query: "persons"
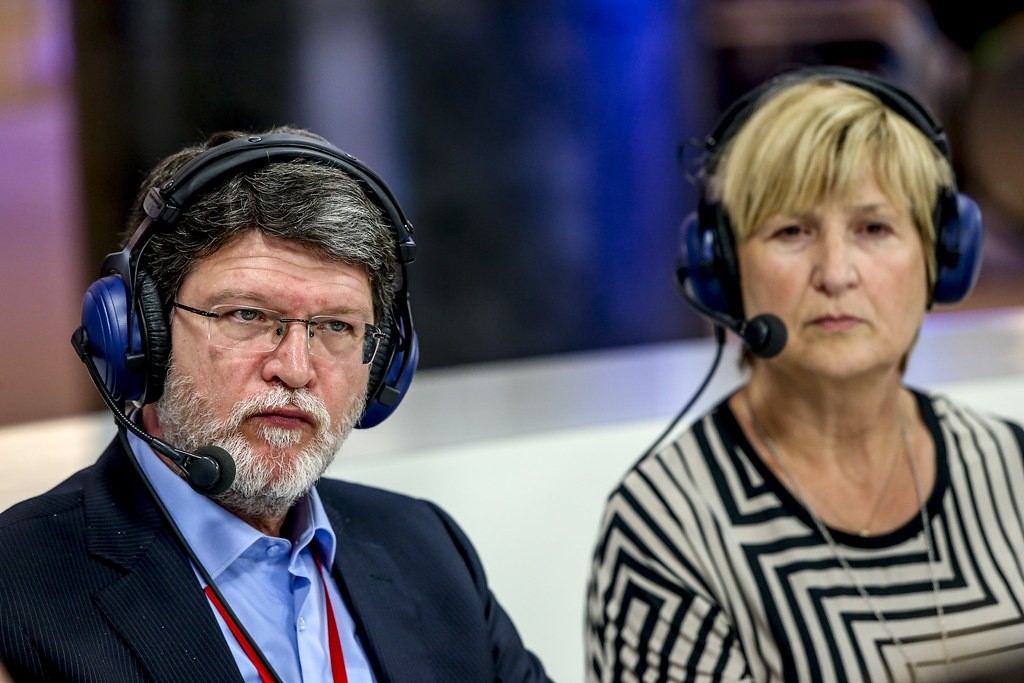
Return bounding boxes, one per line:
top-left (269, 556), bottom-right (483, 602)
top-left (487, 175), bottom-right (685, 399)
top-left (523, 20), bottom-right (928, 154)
top-left (0, 128), bottom-right (555, 683)
top-left (584, 67), bottom-right (1024, 683)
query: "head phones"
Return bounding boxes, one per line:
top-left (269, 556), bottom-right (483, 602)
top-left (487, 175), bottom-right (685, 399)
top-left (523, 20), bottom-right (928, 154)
top-left (678, 63), bottom-right (984, 314)
top-left (71, 131), bottom-right (419, 429)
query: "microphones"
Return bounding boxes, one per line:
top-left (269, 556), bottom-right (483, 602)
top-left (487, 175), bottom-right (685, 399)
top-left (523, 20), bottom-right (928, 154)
top-left (676, 259), bottom-right (787, 361)
top-left (70, 324), bottom-right (236, 496)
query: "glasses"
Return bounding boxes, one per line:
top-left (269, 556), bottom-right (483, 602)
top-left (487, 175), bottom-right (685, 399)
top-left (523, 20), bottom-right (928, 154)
top-left (165, 297), bottom-right (389, 366)
